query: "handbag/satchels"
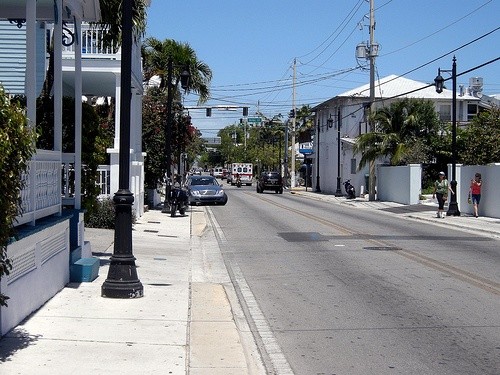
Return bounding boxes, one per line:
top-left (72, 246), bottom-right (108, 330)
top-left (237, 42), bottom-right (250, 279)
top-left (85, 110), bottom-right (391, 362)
top-left (442, 194), bottom-right (448, 201)
top-left (468, 197), bottom-right (471, 203)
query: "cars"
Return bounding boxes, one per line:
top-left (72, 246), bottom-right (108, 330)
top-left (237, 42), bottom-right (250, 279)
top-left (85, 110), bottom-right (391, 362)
top-left (188, 166), bottom-right (222, 178)
top-left (186, 175), bottom-right (228, 205)
top-left (221, 169), bottom-right (229, 180)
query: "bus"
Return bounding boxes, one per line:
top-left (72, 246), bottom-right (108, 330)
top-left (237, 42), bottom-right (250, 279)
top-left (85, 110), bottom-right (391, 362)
top-left (227, 162), bottom-right (253, 186)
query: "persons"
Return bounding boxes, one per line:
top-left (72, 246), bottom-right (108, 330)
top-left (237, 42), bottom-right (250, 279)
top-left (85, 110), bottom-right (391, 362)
top-left (171, 173), bottom-right (189, 217)
top-left (236, 172), bottom-right (242, 182)
top-left (468, 173), bottom-right (483, 218)
top-left (433, 171), bottom-right (455, 218)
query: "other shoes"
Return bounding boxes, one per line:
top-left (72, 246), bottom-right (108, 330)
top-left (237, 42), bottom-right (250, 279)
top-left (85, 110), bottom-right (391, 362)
top-left (441, 214), bottom-right (445, 218)
top-left (474, 212), bottom-right (479, 218)
top-left (436, 213), bottom-right (441, 218)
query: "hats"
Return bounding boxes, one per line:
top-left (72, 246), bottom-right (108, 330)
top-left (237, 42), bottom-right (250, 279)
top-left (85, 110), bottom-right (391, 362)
top-left (438, 172), bottom-right (445, 175)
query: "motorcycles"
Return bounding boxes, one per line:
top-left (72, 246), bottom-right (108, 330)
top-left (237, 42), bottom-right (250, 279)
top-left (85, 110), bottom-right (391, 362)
top-left (168, 182), bottom-right (189, 217)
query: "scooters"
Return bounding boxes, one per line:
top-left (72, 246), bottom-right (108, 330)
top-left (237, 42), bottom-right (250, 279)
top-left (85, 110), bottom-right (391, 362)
top-left (343, 178), bottom-right (356, 199)
top-left (235, 177), bottom-right (242, 188)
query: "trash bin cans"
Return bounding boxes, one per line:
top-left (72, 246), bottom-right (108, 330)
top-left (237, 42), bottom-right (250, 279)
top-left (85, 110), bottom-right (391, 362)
top-left (365, 174), bottom-right (376, 194)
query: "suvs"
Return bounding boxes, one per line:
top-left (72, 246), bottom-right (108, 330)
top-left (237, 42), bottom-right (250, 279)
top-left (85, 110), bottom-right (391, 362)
top-left (256, 171), bottom-right (283, 194)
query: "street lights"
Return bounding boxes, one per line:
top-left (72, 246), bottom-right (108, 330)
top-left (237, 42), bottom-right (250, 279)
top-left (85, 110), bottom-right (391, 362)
top-left (327, 105), bottom-right (342, 199)
top-left (433, 55), bottom-right (461, 217)
